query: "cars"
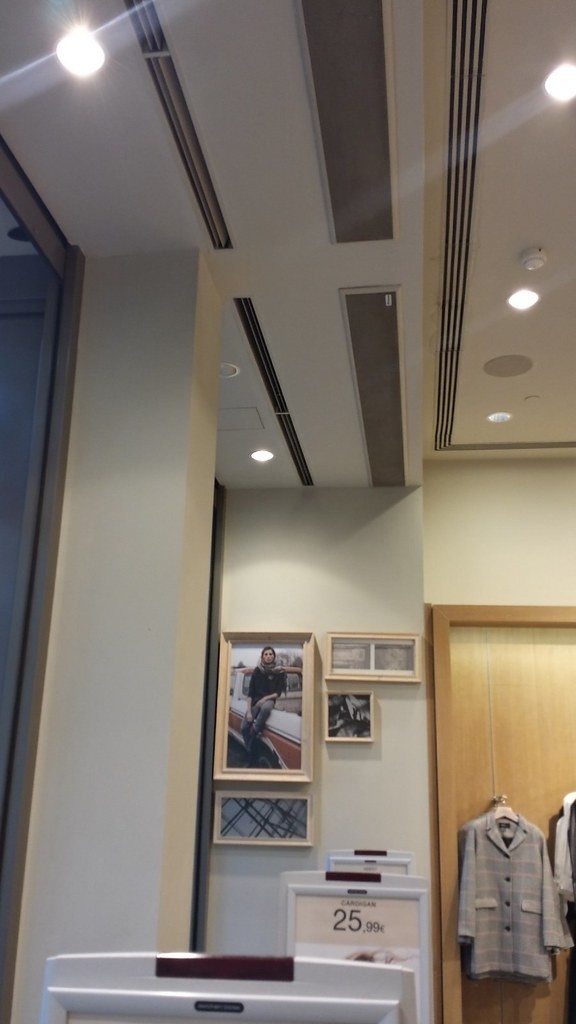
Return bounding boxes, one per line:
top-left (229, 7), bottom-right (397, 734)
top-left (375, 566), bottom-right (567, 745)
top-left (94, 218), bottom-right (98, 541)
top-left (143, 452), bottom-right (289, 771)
top-left (225, 666), bottom-right (304, 769)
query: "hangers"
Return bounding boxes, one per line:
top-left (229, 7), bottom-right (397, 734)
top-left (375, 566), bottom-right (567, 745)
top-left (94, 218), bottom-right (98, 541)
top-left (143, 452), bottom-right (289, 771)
top-left (494, 794), bottom-right (520, 824)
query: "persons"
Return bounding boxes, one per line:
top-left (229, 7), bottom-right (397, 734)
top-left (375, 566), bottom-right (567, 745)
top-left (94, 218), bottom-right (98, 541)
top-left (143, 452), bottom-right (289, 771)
top-left (241, 645), bottom-right (285, 744)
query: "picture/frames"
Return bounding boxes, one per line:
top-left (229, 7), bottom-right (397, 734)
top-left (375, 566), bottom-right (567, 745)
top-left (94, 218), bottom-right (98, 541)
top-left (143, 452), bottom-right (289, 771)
top-left (323, 632), bottom-right (423, 683)
top-left (212, 631), bottom-right (315, 783)
top-left (214, 790), bottom-right (313, 847)
top-left (323, 689), bottom-right (375, 742)
